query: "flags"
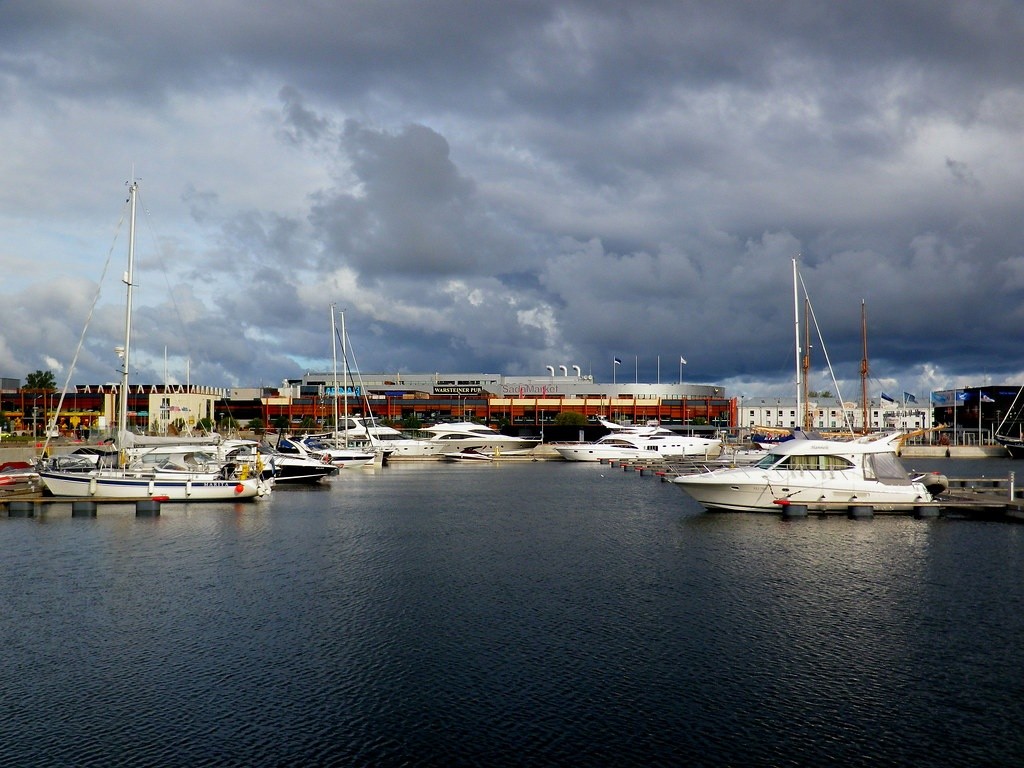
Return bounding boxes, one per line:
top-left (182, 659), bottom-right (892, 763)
top-left (615, 358), bottom-right (621, 364)
top-left (956, 392), bottom-right (970, 400)
top-left (681, 358), bottom-right (687, 364)
top-left (932, 393), bottom-right (948, 403)
top-left (904, 392), bottom-right (918, 404)
top-left (881, 394), bottom-right (894, 406)
top-left (981, 393), bottom-right (995, 402)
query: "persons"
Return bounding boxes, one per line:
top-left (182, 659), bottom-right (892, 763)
top-left (322, 454), bottom-right (332, 464)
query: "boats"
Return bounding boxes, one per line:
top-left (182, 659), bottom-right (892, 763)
top-left (994, 384), bottom-right (1024, 459)
top-left (554, 420), bottom-right (723, 462)
top-left (673, 431), bottom-right (932, 512)
top-left (220, 305), bottom-right (542, 484)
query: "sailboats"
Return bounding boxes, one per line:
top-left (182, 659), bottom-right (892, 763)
top-left (30, 178), bottom-right (276, 501)
